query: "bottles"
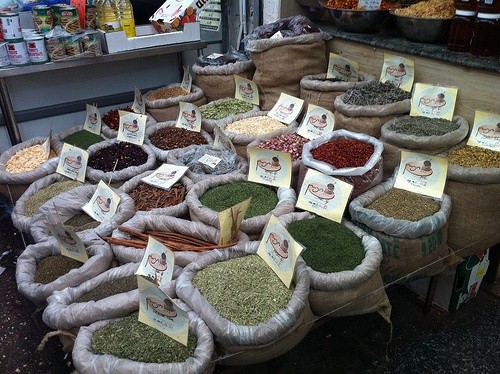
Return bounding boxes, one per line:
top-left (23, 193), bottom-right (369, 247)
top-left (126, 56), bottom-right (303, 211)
top-left (470, 3), bottom-right (500, 54)
top-left (98, 0), bottom-right (137, 37)
top-left (447, 2), bottom-right (477, 51)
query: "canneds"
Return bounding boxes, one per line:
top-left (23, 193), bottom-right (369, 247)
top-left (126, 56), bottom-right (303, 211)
top-left (446, 0), bottom-right (500, 59)
top-left (0, 3), bottom-right (102, 68)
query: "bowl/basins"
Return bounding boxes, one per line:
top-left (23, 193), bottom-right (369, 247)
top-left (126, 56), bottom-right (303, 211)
top-left (296, 1), bottom-right (452, 43)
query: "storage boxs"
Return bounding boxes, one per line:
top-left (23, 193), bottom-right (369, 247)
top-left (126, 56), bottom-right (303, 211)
top-left (148, 0), bottom-right (212, 35)
top-left (401, 248), bottom-right (491, 313)
top-left (97, 20), bottom-right (201, 55)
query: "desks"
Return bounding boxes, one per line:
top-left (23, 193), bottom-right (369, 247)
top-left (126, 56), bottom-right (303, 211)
top-left (0, 38), bottom-right (209, 149)
